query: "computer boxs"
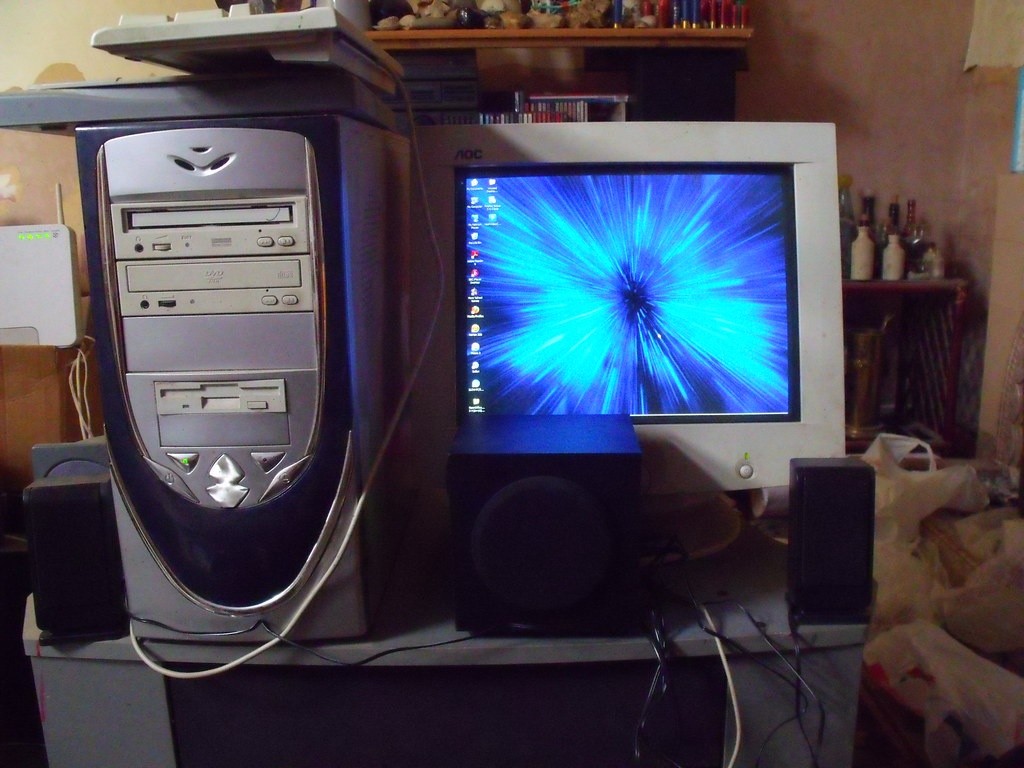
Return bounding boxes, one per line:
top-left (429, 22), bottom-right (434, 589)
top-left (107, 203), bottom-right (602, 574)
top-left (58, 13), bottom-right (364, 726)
top-left (71, 111), bottom-right (411, 641)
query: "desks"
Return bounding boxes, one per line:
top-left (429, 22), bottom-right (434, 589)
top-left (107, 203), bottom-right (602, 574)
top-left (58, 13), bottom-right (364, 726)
top-left (17, 516), bottom-right (871, 768)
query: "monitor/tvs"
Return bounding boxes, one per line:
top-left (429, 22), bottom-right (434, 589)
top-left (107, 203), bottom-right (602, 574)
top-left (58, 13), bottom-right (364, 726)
top-left (411, 119), bottom-right (845, 564)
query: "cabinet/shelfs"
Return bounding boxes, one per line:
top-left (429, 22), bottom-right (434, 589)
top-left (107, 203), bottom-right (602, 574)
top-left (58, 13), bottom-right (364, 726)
top-left (838, 279), bottom-right (969, 452)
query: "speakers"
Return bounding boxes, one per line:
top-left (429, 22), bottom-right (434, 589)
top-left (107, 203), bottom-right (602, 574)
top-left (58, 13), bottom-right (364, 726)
top-left (787, 458), bottom-right (875, 627)
top-left (443, 411), bottom-right (642, 637)
top-left (22, 434), bottom-right (130, 648)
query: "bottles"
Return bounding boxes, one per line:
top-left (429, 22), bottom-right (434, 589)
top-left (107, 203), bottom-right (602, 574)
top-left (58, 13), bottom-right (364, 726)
top-left (839, 175), bottom-right (946, 282)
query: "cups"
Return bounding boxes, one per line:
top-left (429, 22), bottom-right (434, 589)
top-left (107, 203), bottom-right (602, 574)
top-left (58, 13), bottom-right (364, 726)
top-left (846, 326), bottom-right (885, 440)
